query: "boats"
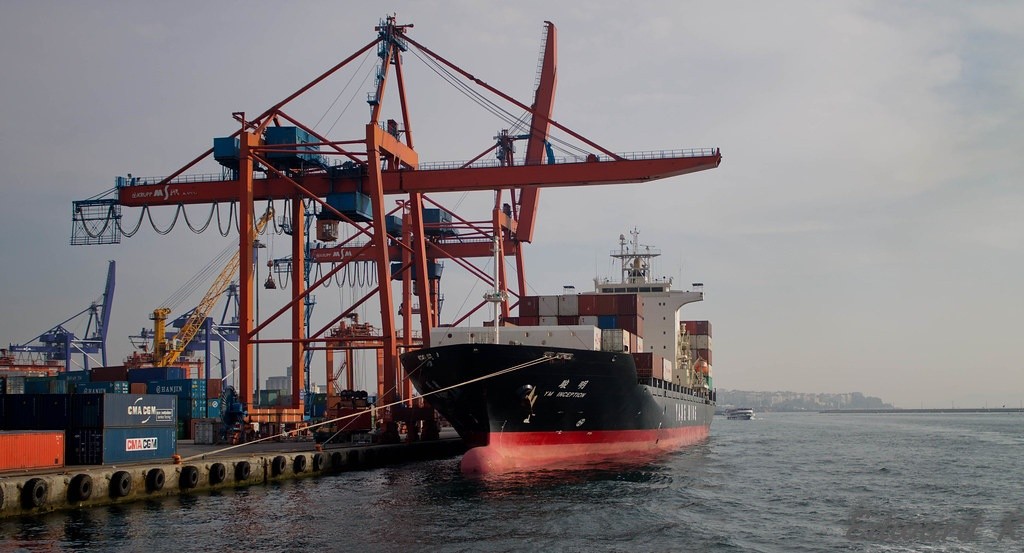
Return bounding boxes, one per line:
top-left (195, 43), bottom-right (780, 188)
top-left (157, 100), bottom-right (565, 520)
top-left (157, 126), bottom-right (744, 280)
top-left (725, 407), bottom-right (753, 421)
top-left (397, 226), bottom-right (719, 476)
